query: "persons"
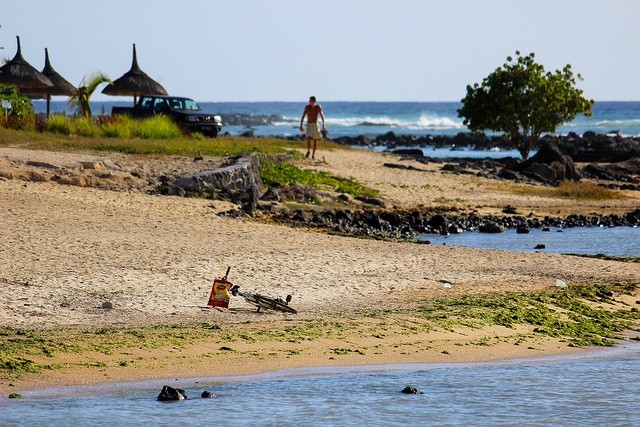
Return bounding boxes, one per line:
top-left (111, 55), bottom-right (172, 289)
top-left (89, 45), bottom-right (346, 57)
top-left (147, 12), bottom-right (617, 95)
top-left (299, 96), bottom-right (326, 159)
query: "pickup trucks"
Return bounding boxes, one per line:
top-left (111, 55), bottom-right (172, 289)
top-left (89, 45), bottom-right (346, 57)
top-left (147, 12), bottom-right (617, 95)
top-left (111, 95), bottom-right (222, 138)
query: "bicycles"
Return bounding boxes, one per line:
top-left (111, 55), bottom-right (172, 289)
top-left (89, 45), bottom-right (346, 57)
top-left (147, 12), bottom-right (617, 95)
top-left (223, 265), bottom-right (298, 314)
